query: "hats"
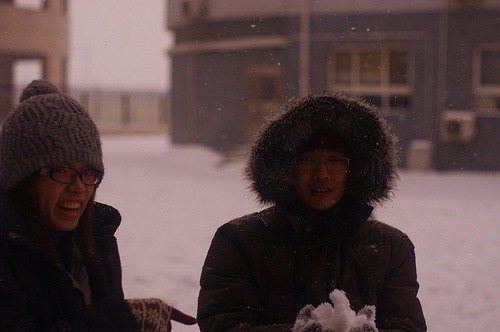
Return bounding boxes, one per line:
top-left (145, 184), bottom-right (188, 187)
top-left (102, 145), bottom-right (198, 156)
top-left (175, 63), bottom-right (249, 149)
top-left (243, 87), bottom-right (399, 209)
top-left (0, 78), bottom-right (105, 193)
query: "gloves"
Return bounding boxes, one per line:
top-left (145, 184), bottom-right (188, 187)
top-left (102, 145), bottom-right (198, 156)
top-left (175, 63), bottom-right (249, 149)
top-left (126, 298), bottom-right (199, 332)
top-left (293, 304), bottom-right (336, 332)
top-left (350, 305), bottom-right (378, 332)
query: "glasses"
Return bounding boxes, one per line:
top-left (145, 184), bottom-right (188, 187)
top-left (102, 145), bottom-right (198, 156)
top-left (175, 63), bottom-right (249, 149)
top-left (43, 166), bottom-right (103, 186)
top-left (293, 157), bottom-right (352, 170)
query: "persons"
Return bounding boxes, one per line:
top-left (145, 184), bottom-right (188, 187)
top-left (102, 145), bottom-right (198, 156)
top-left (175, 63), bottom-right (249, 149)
top-left (196, 89), bottom-right (427, 332)
top-left (0, 79), bottom-right (198, 332)
